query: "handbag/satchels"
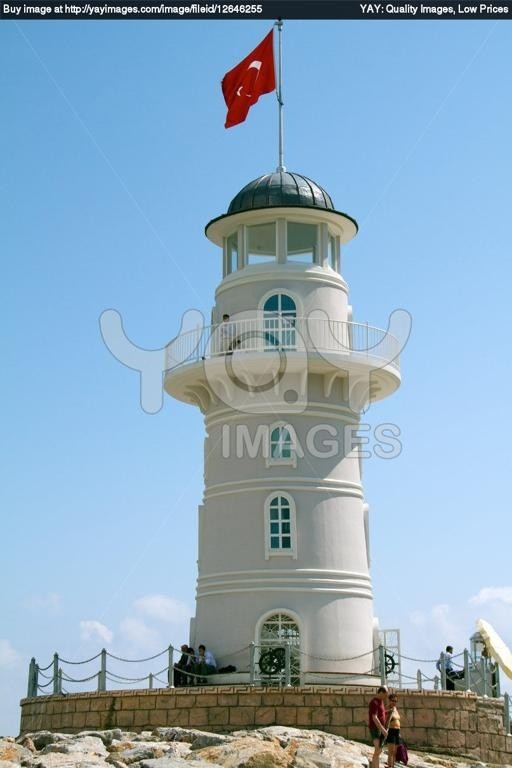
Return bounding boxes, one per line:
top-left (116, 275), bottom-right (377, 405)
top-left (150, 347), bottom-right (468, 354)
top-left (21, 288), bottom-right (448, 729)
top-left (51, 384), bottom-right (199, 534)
top-left (396, 744), bottom-right (408, 766)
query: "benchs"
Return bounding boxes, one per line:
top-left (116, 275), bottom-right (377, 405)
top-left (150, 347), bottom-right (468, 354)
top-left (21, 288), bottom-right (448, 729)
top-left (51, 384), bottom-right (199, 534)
top-left (181, 671), bottom-right (253, 686)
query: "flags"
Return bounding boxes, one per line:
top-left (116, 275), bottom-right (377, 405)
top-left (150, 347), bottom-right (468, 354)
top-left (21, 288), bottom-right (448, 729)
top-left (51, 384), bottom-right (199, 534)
top-left (221, 28), bottom-right (276, 128)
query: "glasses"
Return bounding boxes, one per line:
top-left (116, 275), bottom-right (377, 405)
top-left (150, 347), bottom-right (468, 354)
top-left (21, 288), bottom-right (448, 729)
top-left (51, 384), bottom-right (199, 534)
top-left (391, 699), bottom-right (397, 703)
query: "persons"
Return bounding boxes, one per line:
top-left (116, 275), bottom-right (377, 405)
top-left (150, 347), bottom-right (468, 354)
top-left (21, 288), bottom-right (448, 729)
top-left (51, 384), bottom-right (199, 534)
top-left (174, 644), bottom-right (218, 684)
top-left (368, 685), bottom-right (389, 768)
top-left (436, 646), bottom-right (457, 690)
top-left (384, 694), bottom-right (401, 768)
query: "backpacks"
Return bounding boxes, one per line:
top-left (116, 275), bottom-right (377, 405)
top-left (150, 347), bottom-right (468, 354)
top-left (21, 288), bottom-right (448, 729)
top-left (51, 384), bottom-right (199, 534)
top-left (218, 665), bottom-right (236, 674)
